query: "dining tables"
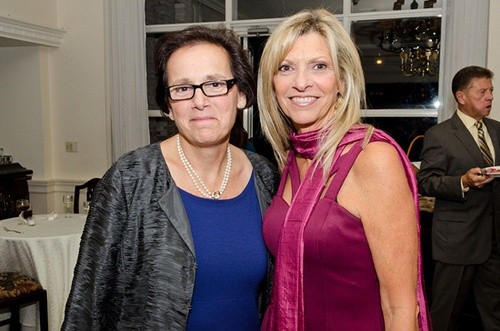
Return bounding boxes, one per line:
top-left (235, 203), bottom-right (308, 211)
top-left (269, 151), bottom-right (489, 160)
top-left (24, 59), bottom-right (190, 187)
top-left (0, 213), bottom-right (91, 331)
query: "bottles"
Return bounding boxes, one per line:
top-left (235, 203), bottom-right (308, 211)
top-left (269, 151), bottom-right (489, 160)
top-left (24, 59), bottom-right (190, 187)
top-left (0, 148), bottom-right (4, 164)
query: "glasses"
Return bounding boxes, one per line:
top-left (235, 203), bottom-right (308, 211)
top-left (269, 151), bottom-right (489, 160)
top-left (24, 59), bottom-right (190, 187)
top-left (165, 78), bottom-right (238, 101)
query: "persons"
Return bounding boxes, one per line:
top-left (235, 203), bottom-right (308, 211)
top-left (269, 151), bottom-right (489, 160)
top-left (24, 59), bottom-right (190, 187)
top-left (255, 8), bottom-right (430, 329)
top-left (414, 64), bottom-right (500, 330)
top-left (61, 25), bottom-right (282, 330)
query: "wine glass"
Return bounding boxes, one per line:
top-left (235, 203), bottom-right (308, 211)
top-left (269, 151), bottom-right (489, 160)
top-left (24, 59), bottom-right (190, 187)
top-left (15, 200), bottom-right (32, 226)
top-left (63, 195), bottom-right (73, 221)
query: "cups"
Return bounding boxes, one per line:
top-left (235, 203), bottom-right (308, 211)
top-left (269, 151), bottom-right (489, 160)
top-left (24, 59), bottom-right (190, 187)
top-left (83, 201), bottom-right (90, 214)
top-left (4, 155), bottom-right (13, 163)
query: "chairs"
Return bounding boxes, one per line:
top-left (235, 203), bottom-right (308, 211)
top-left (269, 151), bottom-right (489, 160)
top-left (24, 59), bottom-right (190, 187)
top-left (0, 271), bottom-right (48, 331)
top-left (74, 177), bottom-right (102, 213)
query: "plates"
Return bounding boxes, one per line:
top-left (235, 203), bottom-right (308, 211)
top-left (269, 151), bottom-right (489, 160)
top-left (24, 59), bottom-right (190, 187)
top-left (484, 166), bottom-right (500, 177)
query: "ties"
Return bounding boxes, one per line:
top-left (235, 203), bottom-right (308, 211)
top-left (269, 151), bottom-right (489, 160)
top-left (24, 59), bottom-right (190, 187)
top-left (473, 120), bottom-right (493, 167)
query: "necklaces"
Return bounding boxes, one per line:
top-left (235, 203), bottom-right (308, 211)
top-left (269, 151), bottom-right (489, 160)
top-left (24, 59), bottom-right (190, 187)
top-left (174, 133), bottom-right (232, 199)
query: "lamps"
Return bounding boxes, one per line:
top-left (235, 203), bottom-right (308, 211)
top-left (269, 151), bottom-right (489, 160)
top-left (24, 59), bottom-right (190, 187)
top-left (355, 0), bottom-right (441, 76)
